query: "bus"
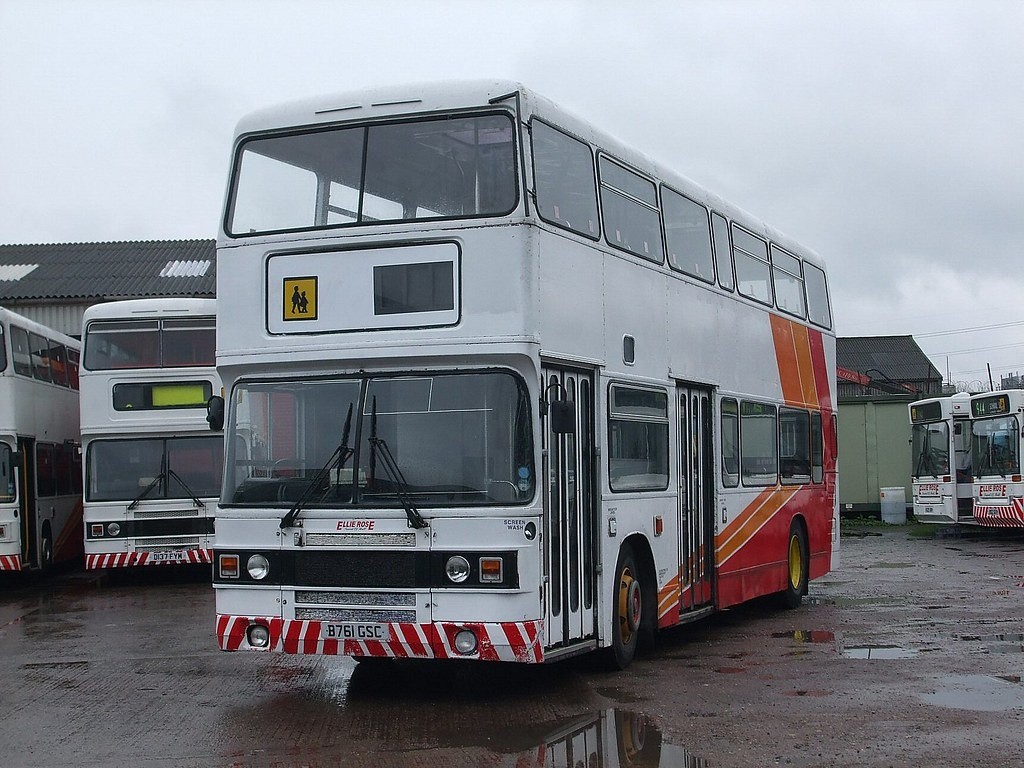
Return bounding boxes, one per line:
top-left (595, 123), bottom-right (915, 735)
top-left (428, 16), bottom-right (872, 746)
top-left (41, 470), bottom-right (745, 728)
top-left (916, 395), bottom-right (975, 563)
top-left (969, 389), bottom-right (1024, 528)
top-left (908, 392), bottom-right (1018, 525)
top-left (212, 78), bottom-right (841, 670)
top-left (0, 307), bottom-right (80, 572)
top-left (78, 298), bottom-right (296, 570)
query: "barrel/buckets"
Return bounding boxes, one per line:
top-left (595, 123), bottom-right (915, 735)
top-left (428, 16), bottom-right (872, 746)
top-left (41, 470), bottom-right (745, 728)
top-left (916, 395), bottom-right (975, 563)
top-left (879, 487), bottom-right (906, 524)
